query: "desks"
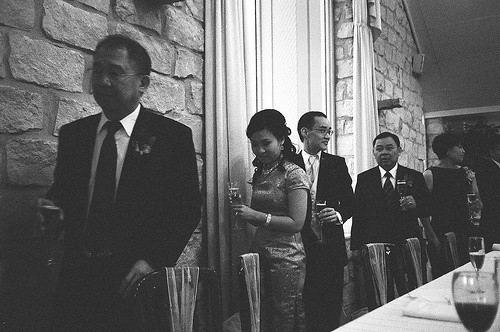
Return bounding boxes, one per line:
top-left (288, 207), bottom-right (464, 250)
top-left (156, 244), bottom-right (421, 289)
top-left (331, 249), bottom-right (500, 332)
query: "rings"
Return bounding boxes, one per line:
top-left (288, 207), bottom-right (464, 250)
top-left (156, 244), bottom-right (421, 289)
top-left (236, 212), bottom-right (238, 215)
top-left (409, 202), bottom-right (412, 205)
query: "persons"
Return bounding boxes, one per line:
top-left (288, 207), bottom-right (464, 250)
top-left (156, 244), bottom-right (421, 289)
top-left (350, 131), bottom-right (432, 311)
top-left (33, 34), bottom-right (204, 332)
top-left (293, 111), bottom-right (355, 332)
top-left (474, 134), bottom-right (500, 256)
top-left (417, 133), bottom-right (484, 279)
top-left (229, 109), bottom-right (313, 332)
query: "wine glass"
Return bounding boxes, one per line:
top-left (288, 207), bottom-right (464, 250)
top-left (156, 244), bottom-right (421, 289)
top-left (466, 194), bottom-right (476, 219)
top-left (468, 237), bottom-right (486, 294)
top-left (314, 199), bottom-right (329, 244)
top-left (34, 192), bottom-right (62, 267)
top-left (227, 182), bottom-right (243, 231)
top-left (396, 179), bottom-right (408, 212)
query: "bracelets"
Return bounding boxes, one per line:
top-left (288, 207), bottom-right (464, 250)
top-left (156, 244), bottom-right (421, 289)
top-left (265, 214), bottom-right (271, 228)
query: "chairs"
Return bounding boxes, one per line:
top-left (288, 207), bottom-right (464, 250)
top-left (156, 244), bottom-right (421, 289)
top-left (134, 232), bottom-right (461, 332)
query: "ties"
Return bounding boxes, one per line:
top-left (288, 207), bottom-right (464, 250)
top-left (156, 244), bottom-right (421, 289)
top-left (85, 120), bottom-right (122, 257)
top-left (306, 156), bottom-right (317, 185)
top-left (384, 172), bottom-right (394, 192)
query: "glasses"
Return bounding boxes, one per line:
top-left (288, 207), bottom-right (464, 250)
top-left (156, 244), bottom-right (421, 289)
top-left (82, 67), bottom-right (137, 82)
top-left (311, 128), bottom-right (334, 136)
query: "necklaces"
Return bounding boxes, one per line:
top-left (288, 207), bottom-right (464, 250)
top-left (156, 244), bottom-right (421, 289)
top-left (262, 164), bottom-right (279, 178)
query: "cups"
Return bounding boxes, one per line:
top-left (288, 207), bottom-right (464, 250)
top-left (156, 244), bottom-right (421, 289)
top-left (452, 271), bottom-right (500, 332)
